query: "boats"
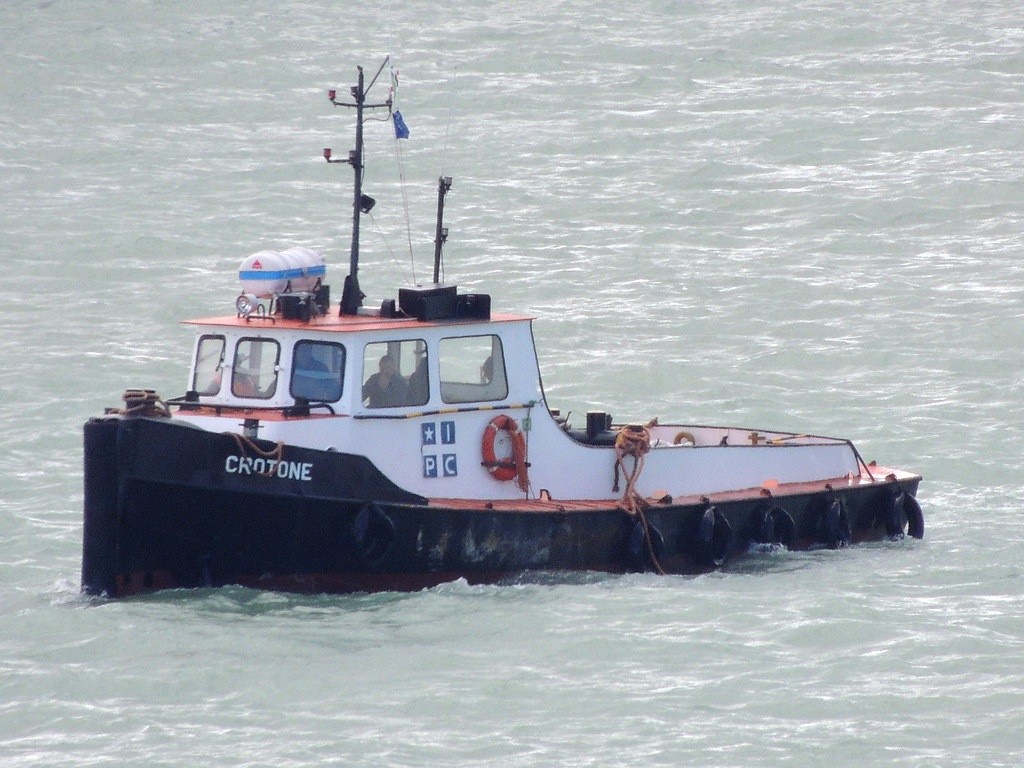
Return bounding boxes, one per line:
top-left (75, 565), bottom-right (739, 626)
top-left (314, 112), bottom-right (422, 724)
top-left (81, 54), bottom-right (926, 603)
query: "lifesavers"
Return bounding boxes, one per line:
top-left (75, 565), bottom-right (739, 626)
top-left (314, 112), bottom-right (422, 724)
top-left (884, 491), bottom-right (925, 541)
top-left (355, 505), bottom-right (400, 568)
top-left (755, 502), bottom-right (798, 551)
top-left (698, 504), bottom-right (737, 568)
top-left (674, 432), bottom-right (697, 445)
top-left (480, 414), bottom-right (526, 481)
top-left (627, 521), bottom-right (666, 575)
top-left (810, 493), bottom-right (853, 547)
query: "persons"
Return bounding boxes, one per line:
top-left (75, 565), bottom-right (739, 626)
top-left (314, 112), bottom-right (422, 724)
top-left (362, 355), bottom-right (407, 408)
top-left (210, 353), bottom-right (260, 398)
top-left (263, 341), bottom-right (339, 400)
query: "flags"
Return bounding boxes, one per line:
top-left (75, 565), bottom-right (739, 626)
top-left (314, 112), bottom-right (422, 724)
top-left (392, 110), bottom-right (410, 139)
